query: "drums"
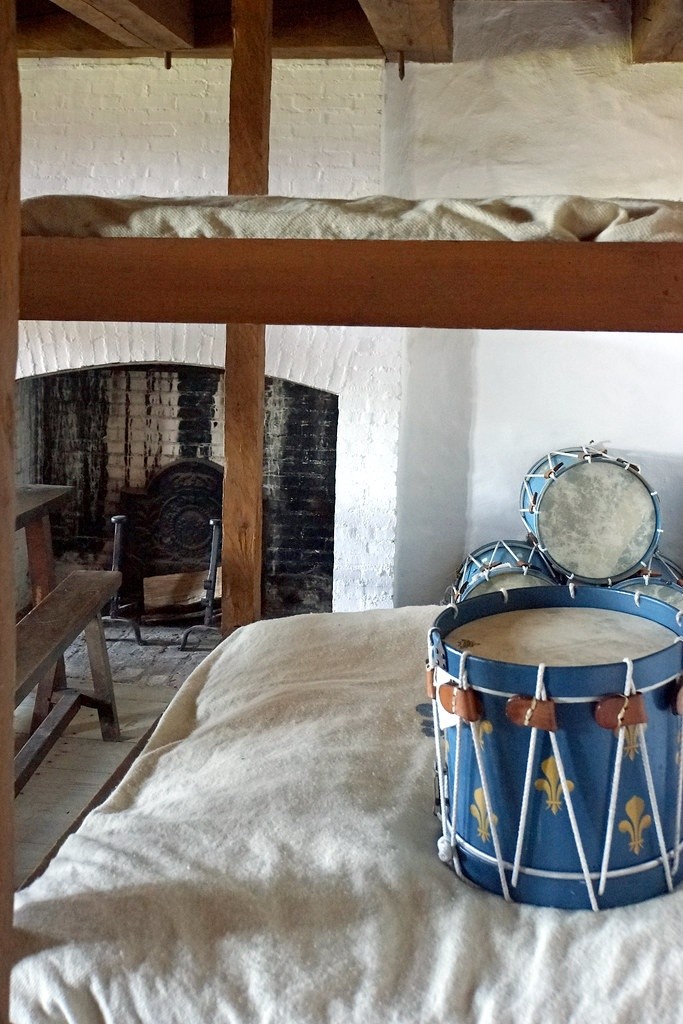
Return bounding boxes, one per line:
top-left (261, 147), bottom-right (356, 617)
top-left (451, 540), bottom-right (564, 602)
top-left (426, 583), bottom-right (683, 909)
top-left (609, 554), bottom-right (683, 612)
top-left (518, 445), bottom-right (663, 584)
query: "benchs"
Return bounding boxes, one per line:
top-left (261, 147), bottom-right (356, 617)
top-left (15, 568), bottom-right (122, 799)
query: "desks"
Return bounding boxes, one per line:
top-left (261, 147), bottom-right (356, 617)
top-left (15, 481), bottom-right (74, 693)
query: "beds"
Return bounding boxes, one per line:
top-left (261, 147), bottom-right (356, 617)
top-left (0, 0), bottom-right (682, 1024)
